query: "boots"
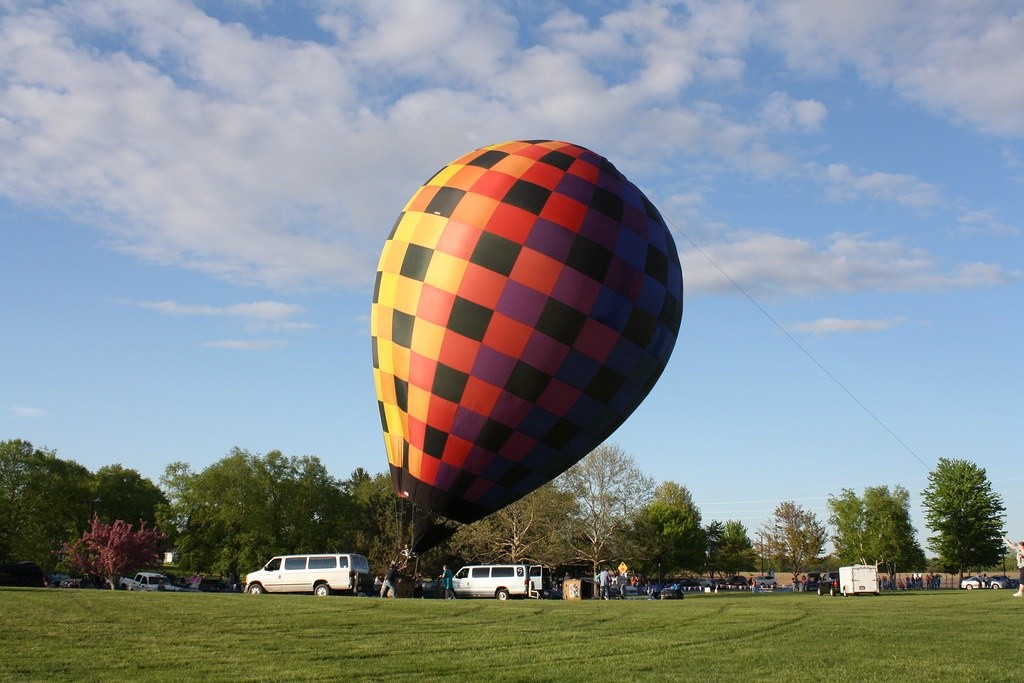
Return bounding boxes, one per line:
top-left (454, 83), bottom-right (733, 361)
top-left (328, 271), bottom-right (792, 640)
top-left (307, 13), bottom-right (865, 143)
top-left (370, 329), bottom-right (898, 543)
top-left (1012, 584), bottom-right (1023, 597)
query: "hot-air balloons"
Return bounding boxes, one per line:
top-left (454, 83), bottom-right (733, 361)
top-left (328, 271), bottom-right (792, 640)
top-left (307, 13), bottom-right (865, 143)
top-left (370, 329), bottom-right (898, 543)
top-left (371, 139), bottom-right (685, 596)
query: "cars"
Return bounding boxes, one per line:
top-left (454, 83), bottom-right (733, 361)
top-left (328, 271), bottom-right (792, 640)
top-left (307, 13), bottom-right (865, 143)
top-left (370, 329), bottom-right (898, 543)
top-left (817, 571), bottom-right (840, 596)
top-left (960, 575), bottom-right (1019, 590)
top-left (612, 575), bottom-right (778, 596)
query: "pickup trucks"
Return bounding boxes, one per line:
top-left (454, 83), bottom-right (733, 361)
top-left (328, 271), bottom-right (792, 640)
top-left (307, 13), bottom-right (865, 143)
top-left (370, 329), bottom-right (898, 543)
top-left (119, 571), bottom-right (181, 592)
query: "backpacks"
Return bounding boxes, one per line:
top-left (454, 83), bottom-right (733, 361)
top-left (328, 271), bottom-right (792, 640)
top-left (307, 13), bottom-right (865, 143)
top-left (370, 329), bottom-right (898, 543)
top-left (595, 574), bottom-right (600, 584)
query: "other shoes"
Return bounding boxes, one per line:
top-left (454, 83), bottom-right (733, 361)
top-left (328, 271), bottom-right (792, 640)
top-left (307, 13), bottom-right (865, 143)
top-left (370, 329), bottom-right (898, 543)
top-left (606, 598), bottom-right (609, 600)
top-left (600, 597), bottom-right (604, 600)
top-left (648, 599), bottom-right (651, 601)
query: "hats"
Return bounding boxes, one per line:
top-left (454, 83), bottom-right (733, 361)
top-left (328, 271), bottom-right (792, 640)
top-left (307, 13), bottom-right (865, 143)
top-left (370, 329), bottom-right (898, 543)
top-left (792, 577), bottom-right (795, 579)
top-left (802, 575), bottom-right (805, 577)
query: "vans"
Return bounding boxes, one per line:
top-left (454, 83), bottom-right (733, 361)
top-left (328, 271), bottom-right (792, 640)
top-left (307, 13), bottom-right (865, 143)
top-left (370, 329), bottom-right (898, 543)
top-left (244, 553), bottom-right (370, 596)
top-left (441, 565), bottom-right (553, 601)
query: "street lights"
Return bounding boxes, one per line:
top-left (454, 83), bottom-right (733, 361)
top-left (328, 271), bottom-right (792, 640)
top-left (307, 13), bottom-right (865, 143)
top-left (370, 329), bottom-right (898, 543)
top-left (754, 531), bottom-right (764, 574)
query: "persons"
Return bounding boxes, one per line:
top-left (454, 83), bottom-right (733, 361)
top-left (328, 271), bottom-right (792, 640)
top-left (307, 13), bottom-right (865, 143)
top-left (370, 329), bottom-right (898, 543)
top-left (68, 570), bottom-right (242, 591)
top-left (545, 537), bottom-right (1024, 601)
top-left (375, 560), bottom-right (456, 599)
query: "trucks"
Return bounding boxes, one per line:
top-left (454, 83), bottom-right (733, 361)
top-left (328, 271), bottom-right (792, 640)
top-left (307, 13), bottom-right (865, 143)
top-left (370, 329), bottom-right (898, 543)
top-left (838, 564), bottom-right (880, 597)
